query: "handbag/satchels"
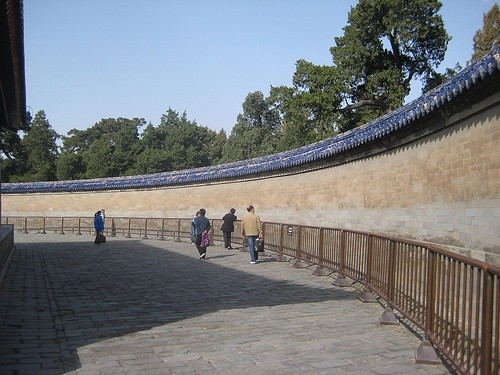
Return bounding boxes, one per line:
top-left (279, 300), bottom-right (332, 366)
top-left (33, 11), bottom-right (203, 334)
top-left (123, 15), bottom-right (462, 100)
top-left (200, 234), bottom-right (210, 248)
top-left (255, 237), bottom-right (265, 252)
top-left (94, 234), bottom-right (107, 244)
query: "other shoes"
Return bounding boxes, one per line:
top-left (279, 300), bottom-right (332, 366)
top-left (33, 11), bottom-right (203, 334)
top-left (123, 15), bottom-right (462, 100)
top-left (201, 257), bottom-right (205, 260)
top-left (226, 246), bottom-right (233, 250)
top-left (250, 260), bottom-right (256, 264)
top-left (200, 253), bottom-right (206, 259)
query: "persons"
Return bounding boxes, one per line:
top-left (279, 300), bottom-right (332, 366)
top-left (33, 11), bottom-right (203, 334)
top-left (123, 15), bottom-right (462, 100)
top-left (191, 208), bottom-right (212, 259)
top-left (241, 205), bottom-right (263, 264)
top-left (94, 209), bottom-right (107, 245)
top-left (221, 208), bottom-right (237, 250)
